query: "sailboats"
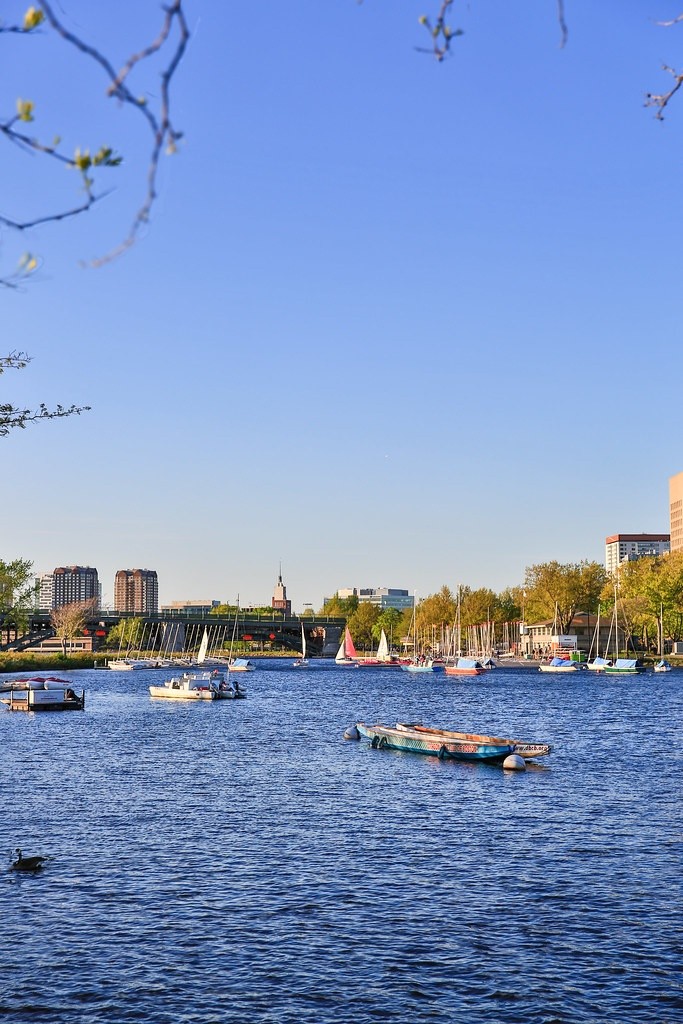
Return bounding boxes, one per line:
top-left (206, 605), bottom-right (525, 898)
top-left (652, 601), bottom-right (672, 674)
top-left (292, 623), bottom-right (309, 667)
top-left (355, 628), bottom-right (415, 666)
top-left (334, 627), bottom-right (360, 666)
top-left (398, 590), bottom-right (442, 672)
top-left (105, 620), bottom-right (236, 671)
top-left (537, 585), bottom-right (647, 674)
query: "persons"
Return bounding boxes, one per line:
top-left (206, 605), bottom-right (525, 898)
top-left (414, 654), bottom-right (429, 667)
top-left (539, 648), bottom-right (542, 654)
top-left (497, 650), bottom-right (500, 659)
top-left (533, 647), bottom-right (536, 654)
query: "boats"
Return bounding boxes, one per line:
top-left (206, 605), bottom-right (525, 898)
top-left (25, 677), bottom-right (48, 689)
top-left (357, 717), bottom-right (512, 762)
top-left (480, 658), bottom-right (497, 670)
top-left (395, 721), bottom-right (554, 763)
top-left (11, 678), bottom-right (31, 690)
top-left (228, 656), bottom-right (257, 671)
top-left (44, 677), bottom-right (74, 691)
top-left (148, 671), bottom-right (249, 700)
top-left (444, 656), bottom-right (487, 676)
